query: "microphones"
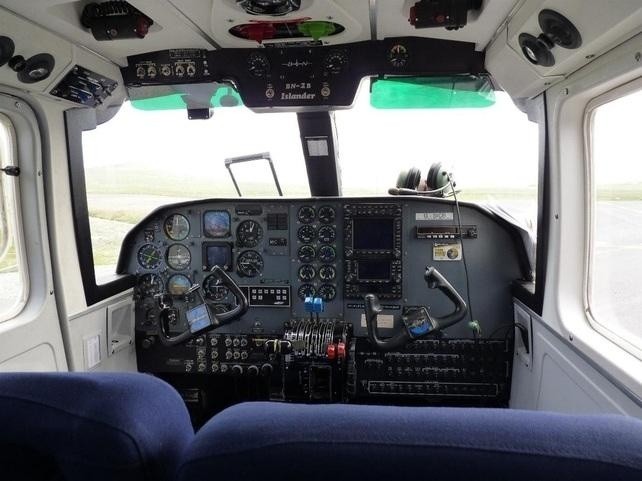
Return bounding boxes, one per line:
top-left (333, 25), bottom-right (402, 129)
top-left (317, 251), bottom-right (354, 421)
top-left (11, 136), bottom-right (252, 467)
top-left (387, 180), bottom-right (451, 195)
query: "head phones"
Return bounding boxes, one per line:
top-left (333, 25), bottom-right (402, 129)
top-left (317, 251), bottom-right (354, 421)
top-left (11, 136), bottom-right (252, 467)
top-left (397, 162), bottom-right (457, 196)
